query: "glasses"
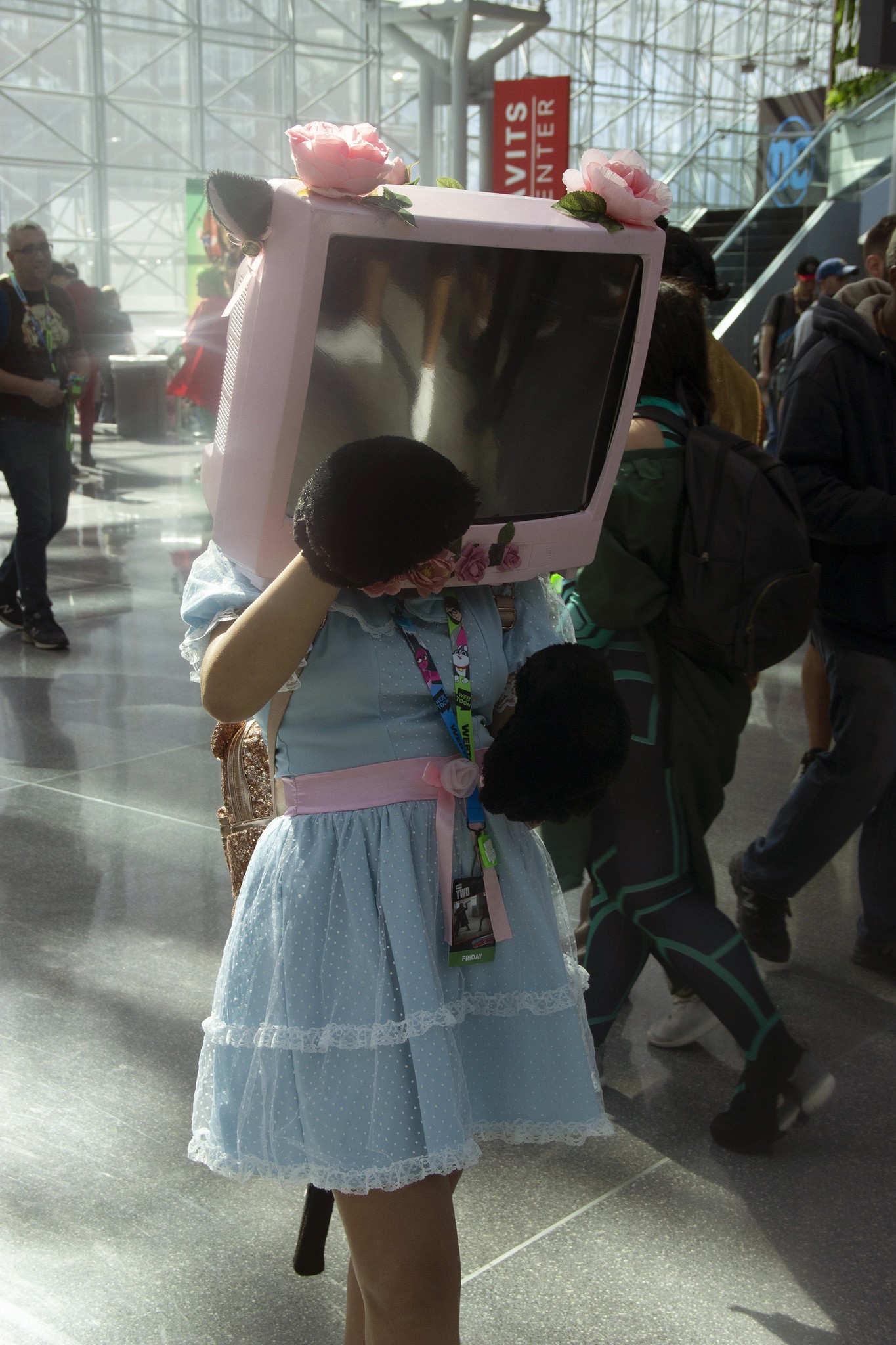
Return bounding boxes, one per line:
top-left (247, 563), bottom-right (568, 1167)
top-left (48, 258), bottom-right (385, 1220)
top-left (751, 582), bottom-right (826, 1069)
top-left (10, 243), bottom-right (53, 255)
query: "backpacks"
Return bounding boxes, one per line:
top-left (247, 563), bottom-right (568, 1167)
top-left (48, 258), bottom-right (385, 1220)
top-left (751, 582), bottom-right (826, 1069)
top-left (772, 334), bottom-right (795, 408)
top-left (751, 331), bottom-right (761, 371)
top-left (211, 582), bottom-right (516, 920)
top-left (633, 404), bottom-right (809, 674)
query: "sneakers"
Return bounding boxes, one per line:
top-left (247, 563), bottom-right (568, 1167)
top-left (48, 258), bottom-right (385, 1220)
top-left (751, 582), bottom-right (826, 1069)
top-left (21, 609), bottom-right (69, 648)
top-left (710, 1049), bottom-right (835, 1151)
top-left (848, 917), bottom-right (895, 972)
top-left (0, 594), bottom-right (23, 629)
top-left (729, 852), bottom-right (792, 963)
top-left (648, 993), bottom-right (721, 1047)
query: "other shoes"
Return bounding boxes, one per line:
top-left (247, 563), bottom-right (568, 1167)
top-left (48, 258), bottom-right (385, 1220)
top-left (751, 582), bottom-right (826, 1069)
top-left (460, 355), bottom-right (512, 434)
top-left (315, 318), bottom-right (382, 366)
top-left (409, 366), bottom-right (434, 444)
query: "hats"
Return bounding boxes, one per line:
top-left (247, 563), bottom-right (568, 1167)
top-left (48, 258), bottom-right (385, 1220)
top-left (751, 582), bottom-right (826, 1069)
top-left (661, 226), bottom-right (728, 301)
top-left (815, 257), bottom-right (859, 283)
top-left (796, 257), bottom-right (820, 282)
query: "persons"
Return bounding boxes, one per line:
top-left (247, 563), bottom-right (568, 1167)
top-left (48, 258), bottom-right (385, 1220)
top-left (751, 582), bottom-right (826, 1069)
top-left (160, 212), bottom-right (896, 1345)
top-left (0, 218), bottom-right (136, 648)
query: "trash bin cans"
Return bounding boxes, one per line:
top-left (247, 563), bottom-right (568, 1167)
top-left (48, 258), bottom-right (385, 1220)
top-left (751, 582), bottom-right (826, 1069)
top-left (108, 354), bottom-right (169, 437)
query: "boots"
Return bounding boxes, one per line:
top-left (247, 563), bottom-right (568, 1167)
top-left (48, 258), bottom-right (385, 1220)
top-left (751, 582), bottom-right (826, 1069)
top-left (68, 450), bottom-right (90, 478)
top-left (81, 441), bottom-right (97, 466)
